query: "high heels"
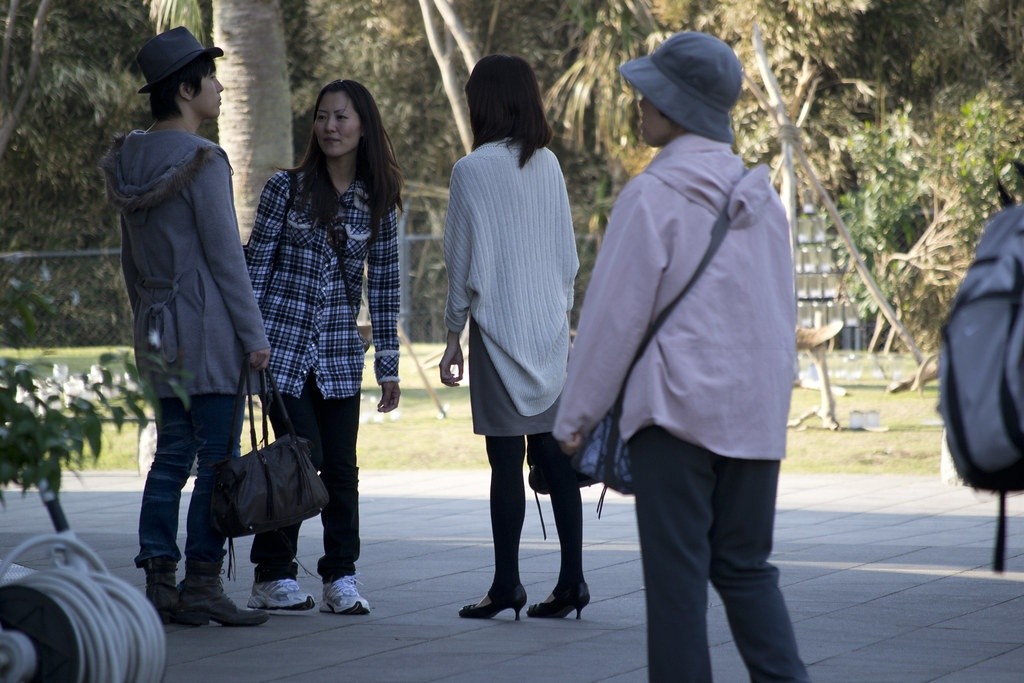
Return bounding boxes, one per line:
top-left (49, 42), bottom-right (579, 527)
top-left (459, 584), bottom-right (527, 620)
top-left (526, 583), bottom-right (590, 619)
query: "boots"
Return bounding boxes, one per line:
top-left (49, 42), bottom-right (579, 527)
top-left (180, 560), bottom-right (269, 625)
top-left (143, 558), bottom-right (209, 625)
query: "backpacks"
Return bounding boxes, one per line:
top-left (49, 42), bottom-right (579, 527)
top-left (939, 158), bottom-right (1024, 572)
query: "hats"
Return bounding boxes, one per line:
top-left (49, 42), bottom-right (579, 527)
top-left (138, 26), bottom-right (224, 94)
top-left (620, 31), bottom-right (743, 143)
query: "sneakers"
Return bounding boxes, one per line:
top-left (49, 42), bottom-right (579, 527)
top-left (248, 578), bottom-right (315, 610)
top-left (320, 572), bottom-right (371, 614)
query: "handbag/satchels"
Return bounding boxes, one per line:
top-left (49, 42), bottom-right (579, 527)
top-left (526, 432), bottom-right (602, 494)
top-left (575, 404), bottom-right (634, 495)
top-left (211, 353), bottom-right (330, 581)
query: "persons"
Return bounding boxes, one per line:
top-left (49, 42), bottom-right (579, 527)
top-left (440, 53), bottom-right (591, 620)
top-left (244, 78), bottom-right (404, 614)
top-left (553, 30), bottom-right (812, 683)
top-left (102, 25), bottom-right (271, 627)
